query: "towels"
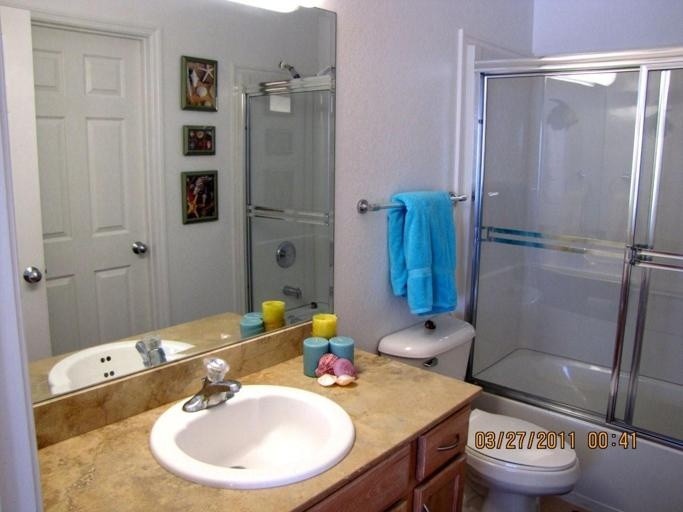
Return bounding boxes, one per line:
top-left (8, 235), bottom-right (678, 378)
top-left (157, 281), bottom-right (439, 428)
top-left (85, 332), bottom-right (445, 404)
top-left (387, 192), bottom-right (458, 317)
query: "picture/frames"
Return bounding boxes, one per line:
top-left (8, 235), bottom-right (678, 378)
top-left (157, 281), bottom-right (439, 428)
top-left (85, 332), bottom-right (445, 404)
top-left (181, 55), bottom-right (219, 112)
top-left (183, 125), bottom-right (216, 155)
top-left (180, 169), bottom-right (219, 225)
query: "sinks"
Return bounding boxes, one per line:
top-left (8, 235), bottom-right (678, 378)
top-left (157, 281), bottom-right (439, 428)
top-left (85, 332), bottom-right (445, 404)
top-left (46, 339), bottom-right (193, 392)
top-left (148, 384), bottom-right (357, 490)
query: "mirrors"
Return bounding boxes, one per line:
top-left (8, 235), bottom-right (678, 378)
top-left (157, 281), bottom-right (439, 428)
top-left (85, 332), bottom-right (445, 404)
top-left (2, 0), bottom-right (336, 408)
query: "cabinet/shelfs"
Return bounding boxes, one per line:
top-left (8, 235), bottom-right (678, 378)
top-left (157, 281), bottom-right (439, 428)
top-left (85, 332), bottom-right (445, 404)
top-left (302, 406), bottom-right (473, 511)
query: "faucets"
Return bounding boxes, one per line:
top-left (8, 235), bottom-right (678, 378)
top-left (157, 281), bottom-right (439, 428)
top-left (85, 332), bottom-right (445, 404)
top-left (183, 356), bottom-right (241, 411)
top-left (135, 336), bottom-right (165, 366)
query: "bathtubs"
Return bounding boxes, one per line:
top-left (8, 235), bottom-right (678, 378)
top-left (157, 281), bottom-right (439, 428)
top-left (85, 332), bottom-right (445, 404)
top-left (468, 347), bottom-right (682, 510)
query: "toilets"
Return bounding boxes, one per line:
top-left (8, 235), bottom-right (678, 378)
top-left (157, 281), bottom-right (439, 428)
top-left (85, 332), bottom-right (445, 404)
top-left (377, 316), bottom-right (582, 512)
